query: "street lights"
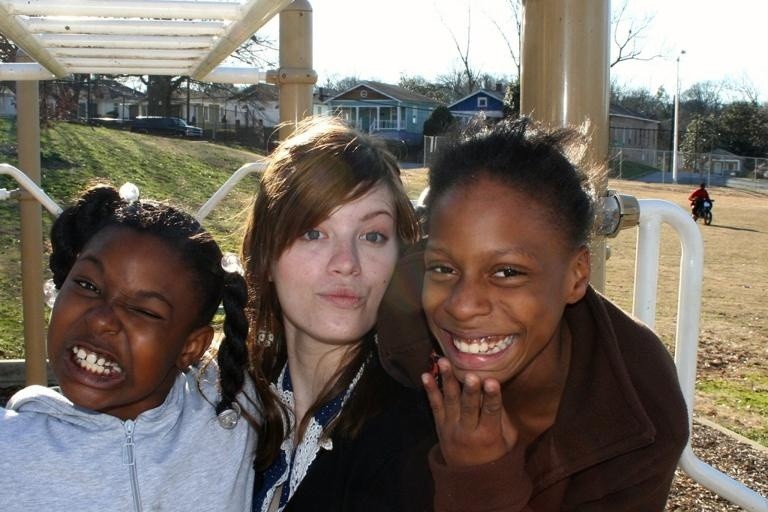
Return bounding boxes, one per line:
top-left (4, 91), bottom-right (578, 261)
top-left (672, 46), bottom-right (691, 184)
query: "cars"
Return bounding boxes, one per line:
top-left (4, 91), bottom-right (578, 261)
top-left (751, 162), bottom-right (767, 179)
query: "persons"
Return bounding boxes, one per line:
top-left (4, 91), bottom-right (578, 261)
top-left (688, 182), bottom-right (709, 219)
top-left (0, 186), bottom-right (263, 510)
top-left (255, 119), bottom-right (265, 149)
top-left (239, 115), bottom-right (437, 511)
top-left (222, 115), bottom-right (227, 123)
top-left (419, 119), bottom-right (690, 510)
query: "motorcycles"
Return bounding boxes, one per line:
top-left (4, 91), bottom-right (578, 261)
top-left (688, 197), bottom-right (715, 225)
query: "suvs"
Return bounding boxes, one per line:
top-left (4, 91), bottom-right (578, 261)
top-left (130, 115), bottom-right (205, 141)
